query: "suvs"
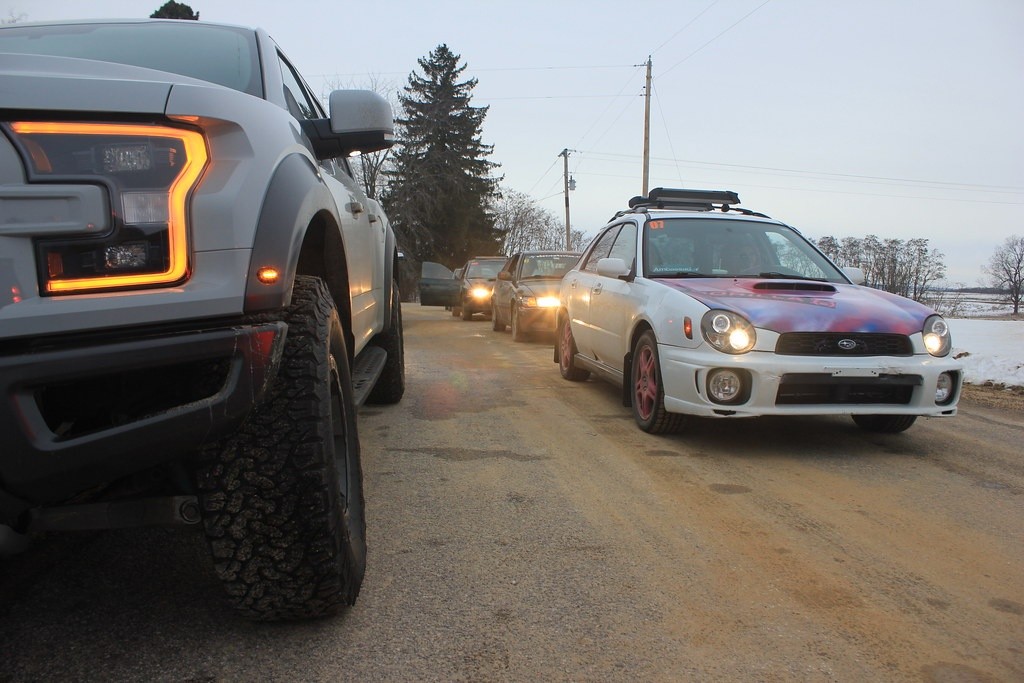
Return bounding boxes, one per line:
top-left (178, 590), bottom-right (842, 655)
top-left (0, 19), bottom-right (407, 625)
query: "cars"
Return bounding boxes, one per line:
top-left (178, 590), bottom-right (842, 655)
top-left (419, 256), bottom-right (509, 321)
top-left (491, 251), bottom-right (582, 342)
top-left (552, 186), bottom-right (963, 436)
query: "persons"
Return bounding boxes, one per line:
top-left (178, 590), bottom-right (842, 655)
top-left (722, 241), bottom-right (767, 274)
top-left (470, 267), bottom-right (482, 276)
top-left (522, 260), bottom-right (537, 275)
top-left (648, 242), bottom-right (663, 269)
